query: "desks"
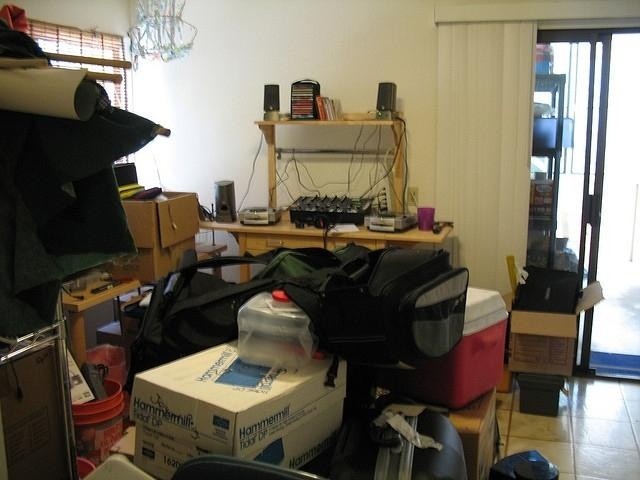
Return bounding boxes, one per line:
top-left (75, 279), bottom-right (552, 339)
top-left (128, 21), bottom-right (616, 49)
top-left (198, 208), bottom-right (457, 287)
top-left (60, 241), bottom-right (227, 370)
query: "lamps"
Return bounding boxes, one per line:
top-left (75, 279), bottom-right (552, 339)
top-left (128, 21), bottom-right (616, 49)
top-left (275, 148), bottom-right (395, 159)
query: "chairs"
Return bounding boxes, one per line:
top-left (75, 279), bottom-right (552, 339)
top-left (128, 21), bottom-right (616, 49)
top-left (170, 455), bottom-right (324, 480)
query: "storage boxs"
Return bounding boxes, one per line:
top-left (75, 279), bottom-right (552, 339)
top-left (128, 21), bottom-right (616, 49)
top-left (95, 320), bottom-right (122, 346)
top-left (129, 337), bottom-right (349, 480)
top-left (108, 427), bottom-right (134, 463)
top-left (0, 340), bottom-right (68, 480)
top-left (507, 280), bottom-right (606, 378)
top-left (526, 75), bottom-right (566, 269)
top-left (443, 385), bottom-right (500, 480)
top-left (112, 292), bottom-right (139, 321)
top-left (415, 286), bottom-right (507, 410)
top-left (104, 190), bottom-right (202, 282)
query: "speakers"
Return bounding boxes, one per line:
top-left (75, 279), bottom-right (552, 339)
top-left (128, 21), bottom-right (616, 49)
top-left (214, 181), bottom-right (237, 223)
top-left (377, 81), bottom-right (397, 109)
top-left (263, 86), bottom-right (280, 109)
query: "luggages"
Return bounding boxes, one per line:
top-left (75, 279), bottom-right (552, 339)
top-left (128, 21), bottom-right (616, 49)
top-left (362, 404), bottom-right (468, 480)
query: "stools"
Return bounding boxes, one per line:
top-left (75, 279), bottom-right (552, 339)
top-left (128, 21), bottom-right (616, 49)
top-left (516, 374), bottom-right (563, 416)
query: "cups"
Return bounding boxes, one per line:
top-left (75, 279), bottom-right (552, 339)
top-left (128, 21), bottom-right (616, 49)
top-left (417, 207), bottom-right (436, 231)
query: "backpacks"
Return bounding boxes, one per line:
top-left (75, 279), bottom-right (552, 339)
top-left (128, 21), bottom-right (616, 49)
top-left (122, 255), bottom-right (276, 397)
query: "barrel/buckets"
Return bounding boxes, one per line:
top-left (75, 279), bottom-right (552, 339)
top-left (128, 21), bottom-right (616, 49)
top-left (71, 379), bottom-right (126, 468)
top-left (86, 343), bottom-right (127, 385)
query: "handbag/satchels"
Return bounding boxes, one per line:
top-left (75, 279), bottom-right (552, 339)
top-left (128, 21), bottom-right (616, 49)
top-left (272, 240), bottom-right (470, 366)
